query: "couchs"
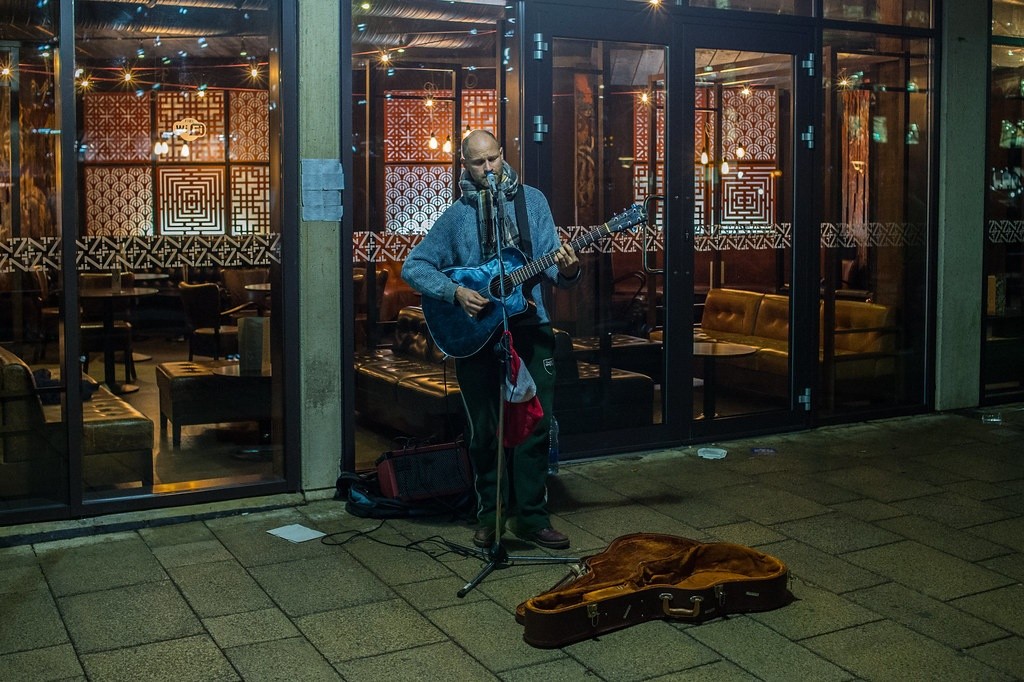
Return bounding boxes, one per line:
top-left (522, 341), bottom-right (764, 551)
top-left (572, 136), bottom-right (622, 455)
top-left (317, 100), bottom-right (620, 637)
top-left (547, 313), bottom-right (653, 429)
top-left (352, 307), bottom-right (471, 445)
top-left (651, 288), bottom-right (900, 417)
top-left (0, 345), bottom-right (154, 499)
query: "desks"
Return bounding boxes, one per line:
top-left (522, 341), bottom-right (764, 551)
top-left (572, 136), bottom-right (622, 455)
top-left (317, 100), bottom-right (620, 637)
top-left (95, 272), bottom-right (170, 363)
top-left (213, 363), bottom-right (273, 462)
top-left (78, 287), bottom-right (160, 396)
top-left (659, 341), bottom-right (756, 416)
top-left (245, 284), bottom-right (270, 293)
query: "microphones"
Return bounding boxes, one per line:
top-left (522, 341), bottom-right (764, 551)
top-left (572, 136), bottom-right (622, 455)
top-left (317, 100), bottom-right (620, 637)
top-left (486, 172), bottom-right (499, 196)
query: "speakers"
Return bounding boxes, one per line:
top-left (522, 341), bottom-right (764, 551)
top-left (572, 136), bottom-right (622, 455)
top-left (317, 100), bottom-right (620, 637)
top-left (375, 440), bottom-right (475, 503)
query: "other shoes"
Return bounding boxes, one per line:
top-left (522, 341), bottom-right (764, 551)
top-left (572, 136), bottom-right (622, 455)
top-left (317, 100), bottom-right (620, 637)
top-left (516, 525), bottom-right (570, 548)
top-left (474, 527), bottom-right (506, 545)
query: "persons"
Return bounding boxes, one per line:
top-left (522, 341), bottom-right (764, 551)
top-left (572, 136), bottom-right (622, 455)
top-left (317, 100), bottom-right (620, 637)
top-left (400, 130), bottom-right (581, 549)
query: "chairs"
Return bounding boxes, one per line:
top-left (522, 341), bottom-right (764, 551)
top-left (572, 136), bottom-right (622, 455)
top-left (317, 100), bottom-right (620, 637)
top-left (607, 261), bottom-right (648, 332)
top-left (179, 281), bottom-right (239, 359)
top-left (352, 268), bottom-right (389, 352)
top-left (33, 267), bottom-right (90, 370)
top-left (838, 259), bottom-right (859, 289)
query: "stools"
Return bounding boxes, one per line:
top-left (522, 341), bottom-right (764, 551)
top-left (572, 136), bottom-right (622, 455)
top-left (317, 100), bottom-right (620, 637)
top-left (572, 335), bottom-right (663, 378)
top-left (157, 360), bottom-right (251, 445)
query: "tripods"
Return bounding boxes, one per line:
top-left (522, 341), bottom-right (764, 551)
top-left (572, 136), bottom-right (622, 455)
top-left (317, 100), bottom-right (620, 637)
top-left (445, 194), bottom-right (582, 598)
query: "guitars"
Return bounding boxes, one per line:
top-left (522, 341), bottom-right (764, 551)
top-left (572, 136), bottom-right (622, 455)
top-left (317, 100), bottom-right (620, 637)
top-left (421, 201), bottom-right (649, 361)
